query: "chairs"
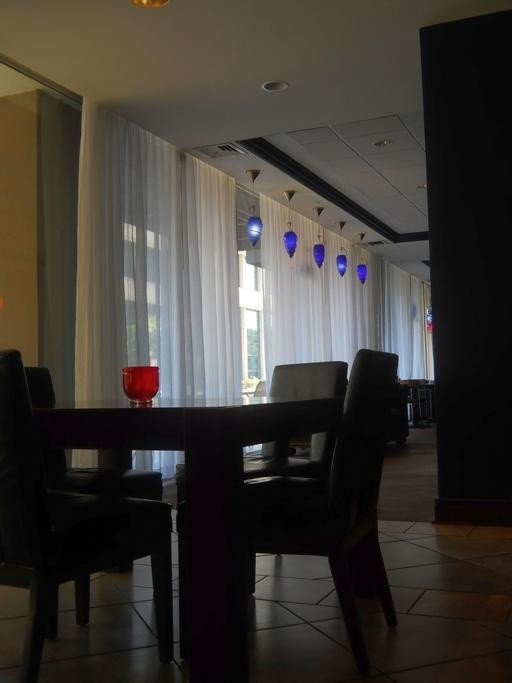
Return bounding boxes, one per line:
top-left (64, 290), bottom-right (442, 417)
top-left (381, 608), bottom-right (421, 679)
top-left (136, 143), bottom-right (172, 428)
top-left (226, 349), bottom-right (397, 675)
top-left (0, 347), bottom-right (175, 683)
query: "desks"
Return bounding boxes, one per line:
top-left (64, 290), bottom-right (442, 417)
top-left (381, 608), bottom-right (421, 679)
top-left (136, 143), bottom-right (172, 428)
top-left (35, 397), bottom-right (342, 682)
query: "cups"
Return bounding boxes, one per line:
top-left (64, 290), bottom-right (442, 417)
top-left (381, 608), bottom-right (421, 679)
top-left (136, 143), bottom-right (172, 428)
top-left (122, 365), bottom-right (160, 403)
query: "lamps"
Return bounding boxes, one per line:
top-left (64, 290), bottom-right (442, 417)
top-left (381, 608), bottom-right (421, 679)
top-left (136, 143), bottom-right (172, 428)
top-left (244, 166), bottom-right (371, 286)
